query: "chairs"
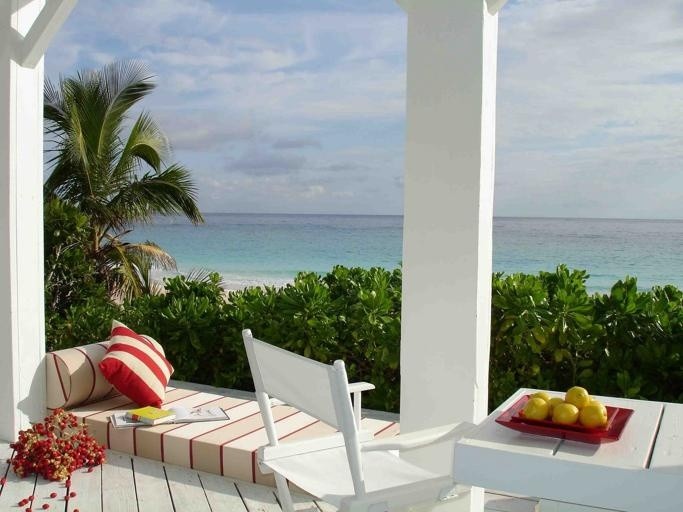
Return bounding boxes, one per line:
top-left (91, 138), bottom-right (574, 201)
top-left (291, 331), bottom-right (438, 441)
top-left (241, 327), bottom-right (485, 512)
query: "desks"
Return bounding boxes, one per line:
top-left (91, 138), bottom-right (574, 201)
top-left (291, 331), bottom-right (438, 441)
top-left (452, 386), bottom-right (683, 512)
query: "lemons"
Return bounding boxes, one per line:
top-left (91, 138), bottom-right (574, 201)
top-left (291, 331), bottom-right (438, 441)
top-left (522, 386), bottom-right (608, 428)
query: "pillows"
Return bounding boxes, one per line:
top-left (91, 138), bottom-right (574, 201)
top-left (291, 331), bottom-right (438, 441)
top-left (45, 332), bottom-right (166, 412)
top-left (98, 317), bottom-right (174, 409)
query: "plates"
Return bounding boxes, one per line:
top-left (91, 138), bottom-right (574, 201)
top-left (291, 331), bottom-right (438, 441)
top-left (496, 393), bottom-right (634, 445)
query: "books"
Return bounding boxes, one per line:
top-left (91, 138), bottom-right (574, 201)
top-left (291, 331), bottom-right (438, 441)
top-left (125, 405), bottom-right (177, 425)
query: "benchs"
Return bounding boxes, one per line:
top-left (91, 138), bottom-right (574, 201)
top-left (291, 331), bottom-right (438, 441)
top-left (65, 390), bottom-right (402, 487)
